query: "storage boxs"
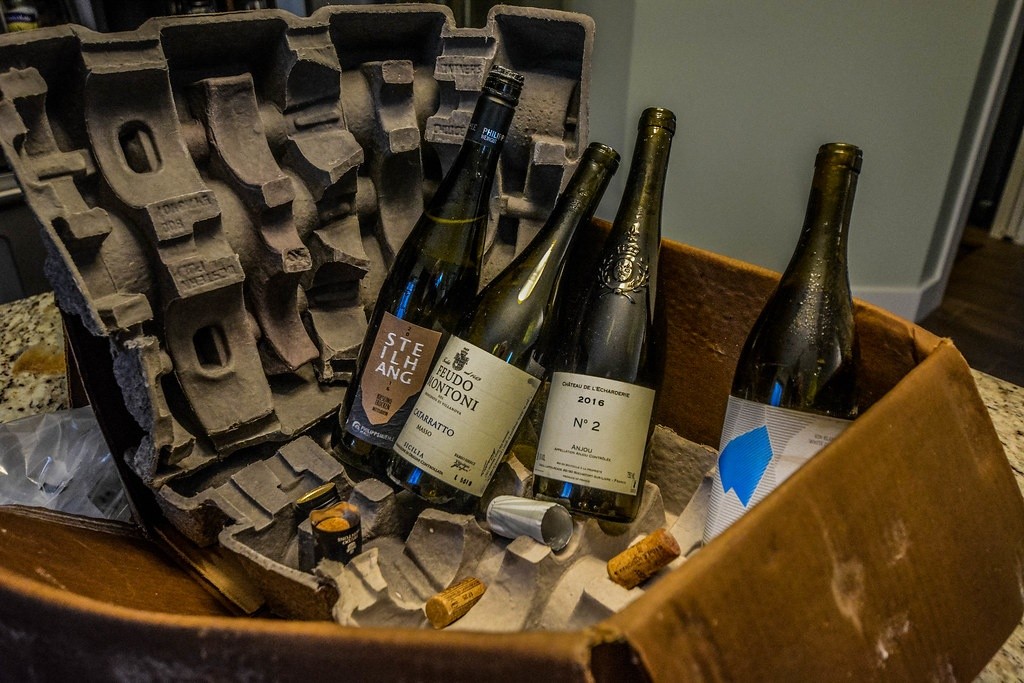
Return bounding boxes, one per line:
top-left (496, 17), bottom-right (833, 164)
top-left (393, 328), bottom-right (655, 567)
top-left (0, 215), bottom-right (1024, 683)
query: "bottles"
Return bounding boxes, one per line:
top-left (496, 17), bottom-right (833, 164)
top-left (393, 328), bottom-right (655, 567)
top-left (385, 142), bottom-right (620, 513)
top-left (701, 143), bottom-right (862, 544)
top-left (328, 64), bottom-right (525, 471)
top-left (533, 105), bottom-right (677, 521)
